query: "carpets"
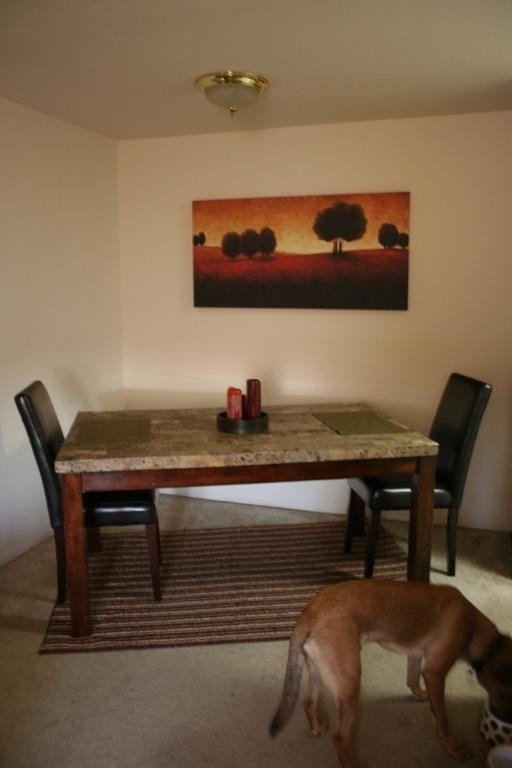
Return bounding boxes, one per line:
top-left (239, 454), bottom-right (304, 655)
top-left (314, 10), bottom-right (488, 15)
top-left (35, 513), bottom-right (429, 656)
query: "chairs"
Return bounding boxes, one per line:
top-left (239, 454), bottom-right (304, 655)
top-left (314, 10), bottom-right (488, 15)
top-left (338, 372), bottom-right (494, 575)
top-left (13, 379), bottom-right (166, 608)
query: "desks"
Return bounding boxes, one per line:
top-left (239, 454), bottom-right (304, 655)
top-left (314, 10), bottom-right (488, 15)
top-left (53, 400), bottom-right (443, 644)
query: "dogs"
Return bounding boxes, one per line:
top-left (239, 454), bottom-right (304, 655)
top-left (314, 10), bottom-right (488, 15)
top-left (262, 576), bottom-right (512, 768)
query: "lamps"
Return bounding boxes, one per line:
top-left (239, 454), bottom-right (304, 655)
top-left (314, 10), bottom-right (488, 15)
top-left (194, 69), bottom-right (269, 121)
